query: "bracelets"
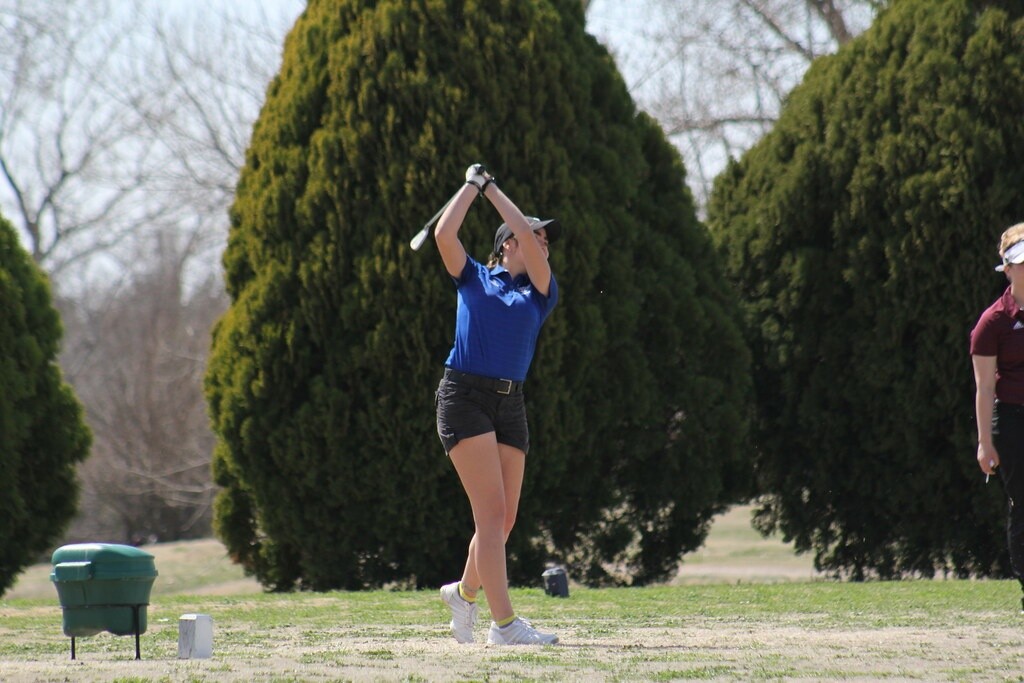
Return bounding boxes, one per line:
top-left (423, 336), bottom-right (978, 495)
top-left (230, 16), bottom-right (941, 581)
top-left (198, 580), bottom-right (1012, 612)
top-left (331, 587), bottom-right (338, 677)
top-left (466, 180), bottom-right (483, 196)
top-left (481, 177), bottom-right (493, 193)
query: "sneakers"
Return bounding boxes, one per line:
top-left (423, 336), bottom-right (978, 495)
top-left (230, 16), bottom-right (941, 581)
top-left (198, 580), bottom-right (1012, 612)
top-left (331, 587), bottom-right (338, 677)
top-left (488, 617), bottom-right (560, 645)
top-left (440, 581), bottom-right (477, 644)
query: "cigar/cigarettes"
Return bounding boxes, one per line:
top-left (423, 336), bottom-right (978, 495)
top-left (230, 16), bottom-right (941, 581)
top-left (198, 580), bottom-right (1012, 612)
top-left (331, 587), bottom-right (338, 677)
top-left (985, 473), bottom-right (990, 484)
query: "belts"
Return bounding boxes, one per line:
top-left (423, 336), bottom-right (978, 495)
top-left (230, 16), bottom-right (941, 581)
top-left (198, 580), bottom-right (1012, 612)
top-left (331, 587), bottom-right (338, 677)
top-left (443, 368), bottom-right (524, 395)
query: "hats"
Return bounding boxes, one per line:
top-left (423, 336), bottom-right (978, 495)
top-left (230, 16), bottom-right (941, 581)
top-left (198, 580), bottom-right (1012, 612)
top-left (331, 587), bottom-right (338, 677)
top-left (995, 241), bottom-right (1024, 272)
top-left (493, 216), bottom-right (561, 254)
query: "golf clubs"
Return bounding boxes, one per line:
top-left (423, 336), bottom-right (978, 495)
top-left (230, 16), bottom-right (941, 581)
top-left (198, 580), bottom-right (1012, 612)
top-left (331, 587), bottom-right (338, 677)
top-left (409, 165), bottom-right (486, 251)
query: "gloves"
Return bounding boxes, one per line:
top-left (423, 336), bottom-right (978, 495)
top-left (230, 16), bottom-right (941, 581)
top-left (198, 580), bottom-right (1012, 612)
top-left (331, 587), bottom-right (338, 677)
top-left (465, 163), bottom-right (488, 187)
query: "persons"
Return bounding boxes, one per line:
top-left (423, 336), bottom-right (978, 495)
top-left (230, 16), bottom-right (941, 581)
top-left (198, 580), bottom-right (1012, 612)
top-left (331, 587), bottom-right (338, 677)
top-left (972, 226), bottom-right (1024, 617)
top-left (433, 164), bottom-right (561, 649)
